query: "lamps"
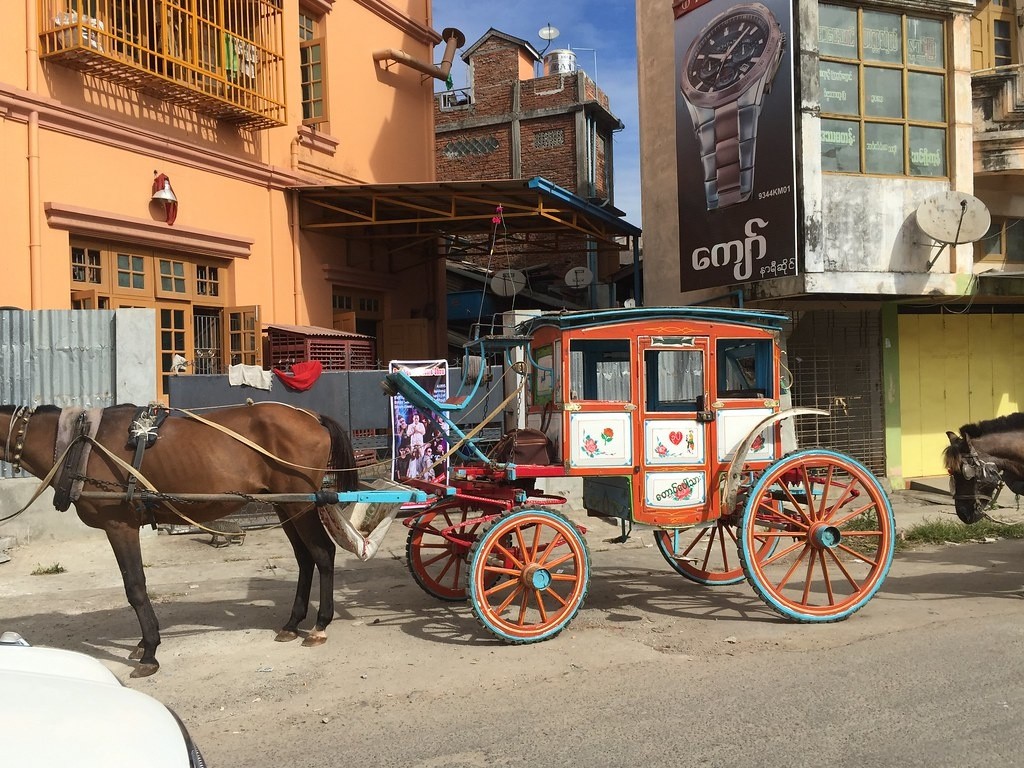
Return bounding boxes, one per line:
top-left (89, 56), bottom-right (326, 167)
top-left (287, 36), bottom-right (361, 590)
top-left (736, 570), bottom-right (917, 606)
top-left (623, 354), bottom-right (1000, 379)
top-left (151, 179), bottom-right (177, 204)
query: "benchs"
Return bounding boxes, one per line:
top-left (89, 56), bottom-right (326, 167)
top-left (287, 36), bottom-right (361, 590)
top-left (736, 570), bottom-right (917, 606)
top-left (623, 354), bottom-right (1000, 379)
top-left (696, 388), bottom-right (766, 411)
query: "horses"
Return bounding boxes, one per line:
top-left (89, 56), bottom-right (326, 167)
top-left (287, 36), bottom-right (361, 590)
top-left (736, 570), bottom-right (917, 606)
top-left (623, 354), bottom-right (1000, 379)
top-left (942, 413), bottom-right (1024, 525)
top-left (0, 403), bottom-right (358, 678)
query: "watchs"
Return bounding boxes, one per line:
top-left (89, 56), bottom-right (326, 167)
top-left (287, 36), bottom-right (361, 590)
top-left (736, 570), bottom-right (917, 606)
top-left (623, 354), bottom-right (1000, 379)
top-left (680, 3), bottom-right (786, 209)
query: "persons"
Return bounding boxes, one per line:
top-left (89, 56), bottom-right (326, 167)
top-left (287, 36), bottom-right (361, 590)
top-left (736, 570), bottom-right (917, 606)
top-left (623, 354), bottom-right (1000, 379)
top-left (396, 413), bottom-right (446, 483)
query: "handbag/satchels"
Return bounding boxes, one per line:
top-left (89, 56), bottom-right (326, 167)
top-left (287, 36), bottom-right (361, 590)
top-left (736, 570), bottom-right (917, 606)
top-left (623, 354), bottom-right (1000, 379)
top-left (494, 427), bottom-right (554, 465)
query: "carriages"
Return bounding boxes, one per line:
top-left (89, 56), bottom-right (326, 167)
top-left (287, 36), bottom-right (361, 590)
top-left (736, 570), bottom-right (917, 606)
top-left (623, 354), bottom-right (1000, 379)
top-left (1, 304), bottom-right (898, 646)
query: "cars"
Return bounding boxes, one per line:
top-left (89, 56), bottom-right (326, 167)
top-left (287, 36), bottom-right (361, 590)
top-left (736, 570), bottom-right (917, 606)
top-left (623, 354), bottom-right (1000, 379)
top-left (1, 628), bottom-right (208, 768)
